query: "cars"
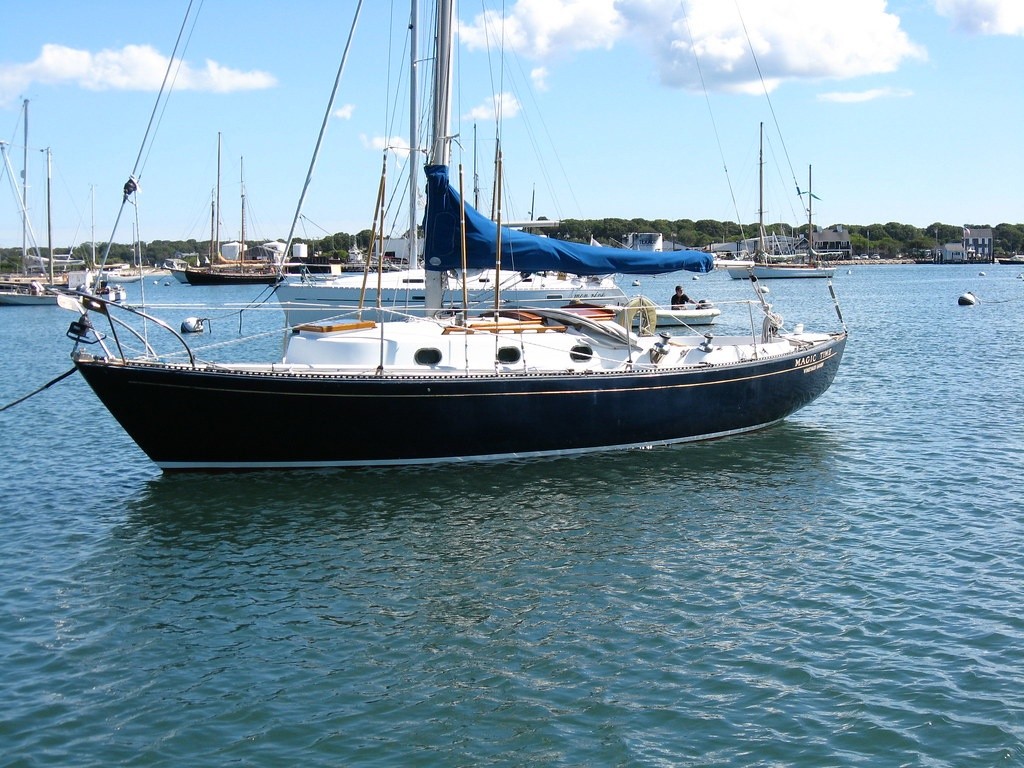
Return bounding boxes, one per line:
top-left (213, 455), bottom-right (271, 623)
top-left (860, 254), bottom-right (868, 259)
top-left (871, 254), bottom-right (880, 259)
top-left (852, 255), bottom-right (860, 260)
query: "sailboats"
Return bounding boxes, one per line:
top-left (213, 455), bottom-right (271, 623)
top-left (0, 97), bottom-right (128, 306)
top-left (70, 0), bottom-right (846, 473)
top-left (160, 132), bottom-right (287, 287)
top-left (60, 185), bottom-right (144, 284)
top-left (726, 124), bottom-right (836, 281)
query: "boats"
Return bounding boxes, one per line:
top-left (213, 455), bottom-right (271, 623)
top-left (998, 256), bottom-right (1024, 265)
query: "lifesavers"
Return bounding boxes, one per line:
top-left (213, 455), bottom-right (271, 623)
top-left (619, 297), bottom-right (657, 337)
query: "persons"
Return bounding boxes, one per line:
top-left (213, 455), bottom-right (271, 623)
top-left (671, 285), bottom-right (701, 310)
